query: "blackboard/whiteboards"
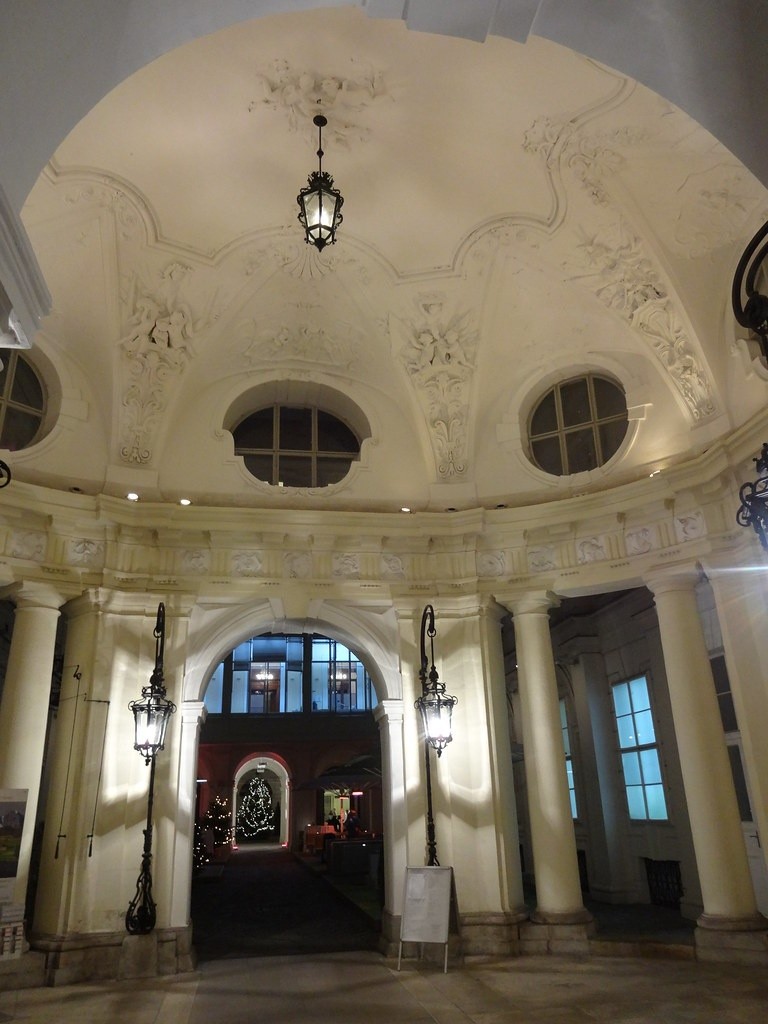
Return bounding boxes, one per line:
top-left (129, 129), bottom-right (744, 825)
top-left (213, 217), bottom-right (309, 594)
top-left (399, 866), bottom-right (455, 944)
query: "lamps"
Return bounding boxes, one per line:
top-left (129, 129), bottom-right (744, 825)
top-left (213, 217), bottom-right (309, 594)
top-left (412, 605), bottom-right (458, 867)
top-left (297, 110), bottom-right (344, 254)
top-left (125, 599), bottom-right (175, 936)
